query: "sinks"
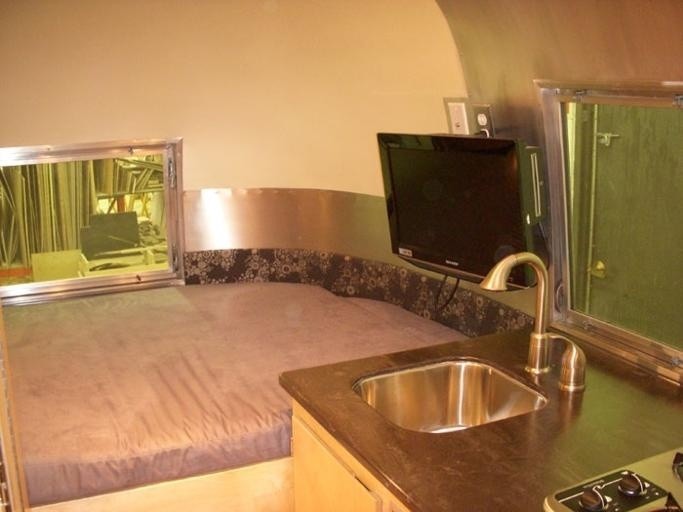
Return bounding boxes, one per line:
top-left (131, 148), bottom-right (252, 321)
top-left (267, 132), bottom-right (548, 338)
top-left (352, 357), bottom-right (548, 434)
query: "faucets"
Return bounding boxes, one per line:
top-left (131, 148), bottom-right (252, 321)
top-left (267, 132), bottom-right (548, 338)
top-left (479, 251), bottom-right (553, 375)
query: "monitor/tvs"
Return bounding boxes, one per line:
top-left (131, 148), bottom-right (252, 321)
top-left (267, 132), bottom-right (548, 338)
top-left (377, 131), bottom-right (553, 291)
